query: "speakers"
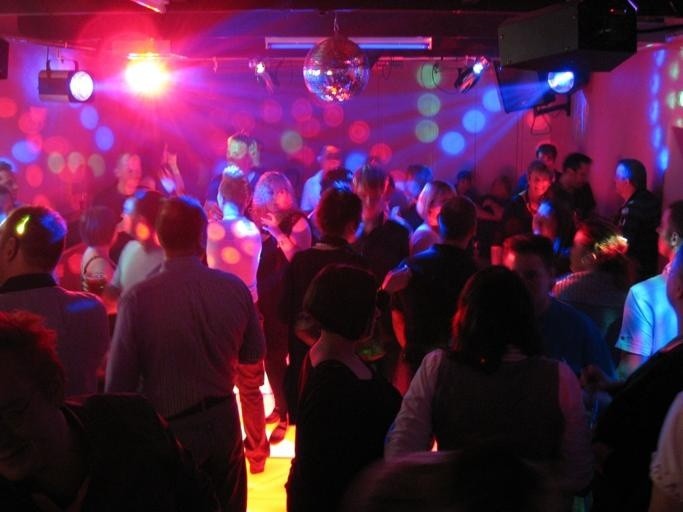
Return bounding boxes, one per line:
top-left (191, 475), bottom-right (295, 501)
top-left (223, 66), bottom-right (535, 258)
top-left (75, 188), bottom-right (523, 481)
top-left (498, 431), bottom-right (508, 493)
top-left (497, 0), bottom-right (638, 73)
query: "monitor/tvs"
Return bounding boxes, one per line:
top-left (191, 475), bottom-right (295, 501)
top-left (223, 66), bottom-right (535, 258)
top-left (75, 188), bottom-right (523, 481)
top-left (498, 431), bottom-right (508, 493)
top-left (493, 60), bottom-right (555, 114)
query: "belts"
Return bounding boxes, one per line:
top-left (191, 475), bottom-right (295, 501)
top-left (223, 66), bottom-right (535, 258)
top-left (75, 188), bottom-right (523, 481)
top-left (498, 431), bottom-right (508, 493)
top-left (164, 392), bottom-right (236, 422)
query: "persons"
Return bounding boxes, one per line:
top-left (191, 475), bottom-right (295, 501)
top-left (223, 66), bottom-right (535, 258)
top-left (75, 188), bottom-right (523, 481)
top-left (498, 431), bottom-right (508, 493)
top-left (0, 131), bottom-right (682, 512)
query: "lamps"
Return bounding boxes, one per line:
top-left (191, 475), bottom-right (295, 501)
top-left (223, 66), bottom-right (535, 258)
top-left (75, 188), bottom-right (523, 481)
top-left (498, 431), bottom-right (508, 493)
top-left (545, 69), bottom-right (592, 95)
top-left (249, 59), bottom-right (267, 76)
top-left (126, 42), bottom-right (170, 101)
top-left (301, 14), bottom-right (370, 106)
top-left (452, 57), bottom-right (488, 93)
top-left (261, 33), bottom-right (434, 54)
top-left (37, 45), bottom-right (93, 104)
